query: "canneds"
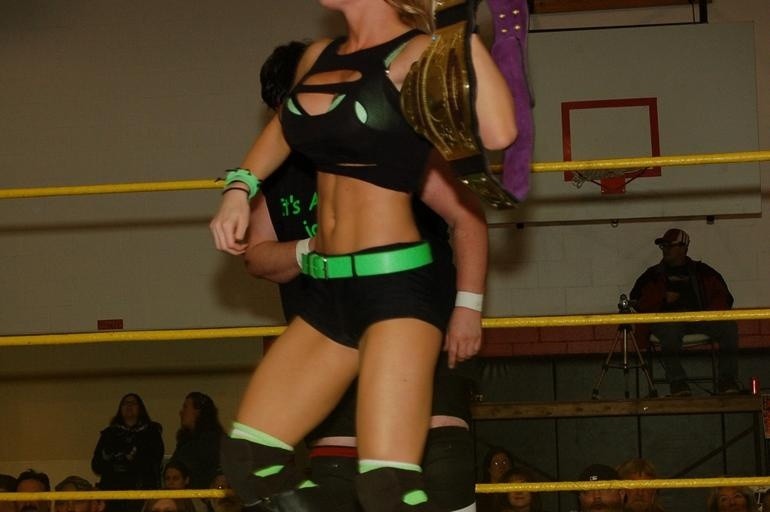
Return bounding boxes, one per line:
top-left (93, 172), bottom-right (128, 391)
top-left (751, 376), bottom-right (760, 395)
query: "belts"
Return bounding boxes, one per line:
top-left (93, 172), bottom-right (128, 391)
top-left (300, 241), bottom-right (434, 279)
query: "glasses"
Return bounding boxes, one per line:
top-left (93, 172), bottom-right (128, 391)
top-left (493, 460), bottom-right (509, 466)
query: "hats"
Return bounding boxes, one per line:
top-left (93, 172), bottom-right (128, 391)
top-left (653, 228), bottom-right (690, 246)
top-left (577, 464), bottom-right (618, 482)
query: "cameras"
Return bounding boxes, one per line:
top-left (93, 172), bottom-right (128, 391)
top-left (618, 294), bottom-right (637, 313)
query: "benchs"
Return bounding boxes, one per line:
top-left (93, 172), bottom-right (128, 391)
top-left (468, 309), bottom-right (768, 356)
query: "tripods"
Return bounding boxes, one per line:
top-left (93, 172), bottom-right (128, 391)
top-left (592, 325), bottom-right (658, 400)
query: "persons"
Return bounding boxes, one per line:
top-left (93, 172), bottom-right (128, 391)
top-left (629, 229), bottom-right (740, 396)
